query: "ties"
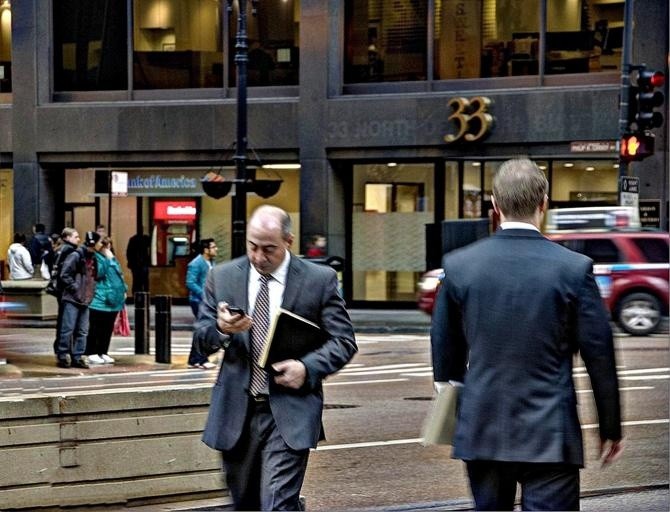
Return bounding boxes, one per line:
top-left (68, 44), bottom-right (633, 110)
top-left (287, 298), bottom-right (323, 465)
top-left (248, 274), bottom-right (273, 398)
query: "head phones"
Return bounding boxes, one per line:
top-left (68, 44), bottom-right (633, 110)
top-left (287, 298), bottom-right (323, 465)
top-left (87, 231), bottom-right (96, 247)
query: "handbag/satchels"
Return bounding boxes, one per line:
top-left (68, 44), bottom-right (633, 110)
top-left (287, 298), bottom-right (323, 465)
top-left (113, 304), bottom-right (131, 336)
top-left (46, 265), bottom-right (64, 296)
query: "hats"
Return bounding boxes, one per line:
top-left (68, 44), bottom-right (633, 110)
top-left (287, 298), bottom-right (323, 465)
top-left (86, 232), bottom-right (100, 246)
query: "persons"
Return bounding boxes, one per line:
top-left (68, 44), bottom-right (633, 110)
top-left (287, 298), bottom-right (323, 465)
top-left (193, 203), bottom-right (359, 511)
top-left (187, 237), bottom-right (219, 369)
top-left (306, 234), bottom-right (328, 257)
top-left (429, 156), bottom-right (627, 511)
top-left (1, 220), bottom-right (150, 369)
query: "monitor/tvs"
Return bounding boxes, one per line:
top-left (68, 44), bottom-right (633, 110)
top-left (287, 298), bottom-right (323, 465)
top-left (174, 244), bottom-right (185, 256)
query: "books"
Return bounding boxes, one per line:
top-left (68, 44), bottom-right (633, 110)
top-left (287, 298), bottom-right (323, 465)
top-left (419, 379), bottom-right (465, 448)
top-left (252, 304), bottom-right (323, 376)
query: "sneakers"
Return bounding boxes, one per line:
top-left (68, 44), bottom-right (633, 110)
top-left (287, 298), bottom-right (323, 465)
top-left (188, 362), bottom-right (217, 370)
top-left (101, 354), bottom-right (115, 363)
top-left (58, 360), bottom-right (88, 368)
top-left (87, 354), bottom-right (104, 364)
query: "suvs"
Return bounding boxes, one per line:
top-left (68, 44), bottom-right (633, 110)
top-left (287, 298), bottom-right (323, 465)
top-left (416, 228), bottom-right (669, 337)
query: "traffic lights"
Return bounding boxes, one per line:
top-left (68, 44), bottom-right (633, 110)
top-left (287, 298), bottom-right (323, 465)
top-left (619, 63), bottom-right (667, 162)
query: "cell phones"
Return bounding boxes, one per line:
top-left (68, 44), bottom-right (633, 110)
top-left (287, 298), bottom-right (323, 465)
top-left (226, 306), bottom-right (244, 320)
